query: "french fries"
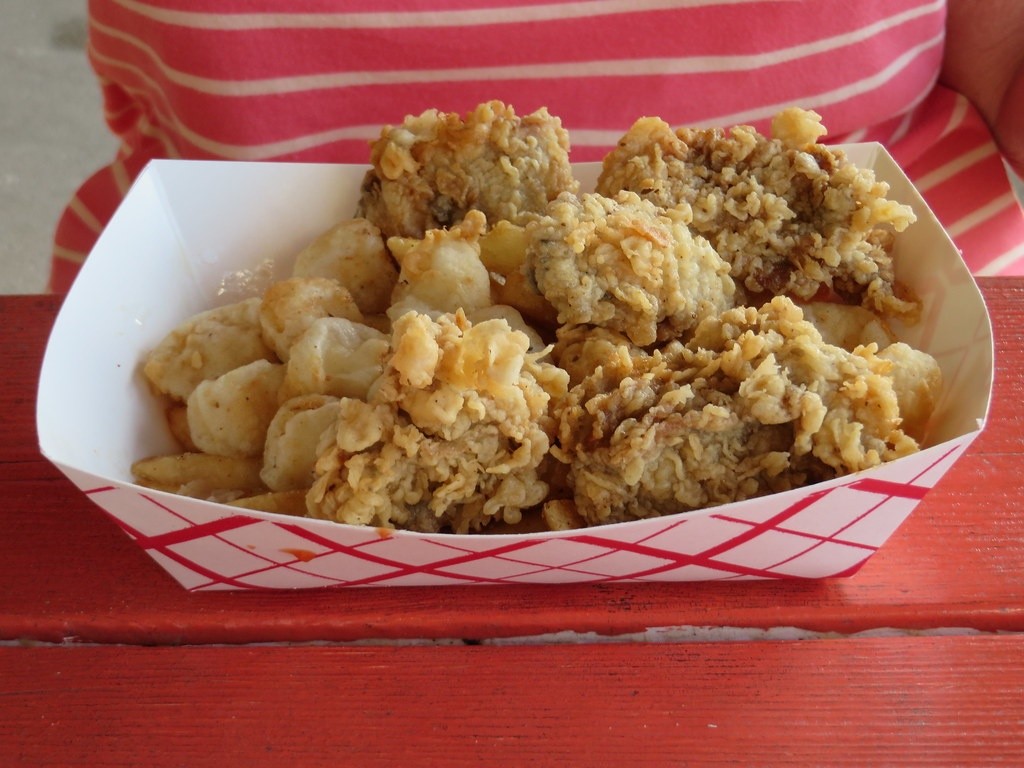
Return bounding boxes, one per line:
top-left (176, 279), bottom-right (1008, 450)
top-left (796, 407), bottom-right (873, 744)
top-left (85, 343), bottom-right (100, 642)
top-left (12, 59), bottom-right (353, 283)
top-left (134, 404), bottom-right (310, 518)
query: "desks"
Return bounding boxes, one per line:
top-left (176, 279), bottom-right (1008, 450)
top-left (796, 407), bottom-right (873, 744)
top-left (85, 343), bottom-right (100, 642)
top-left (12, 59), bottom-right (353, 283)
top-left (1, 276), bottom-right (1023, 768)
